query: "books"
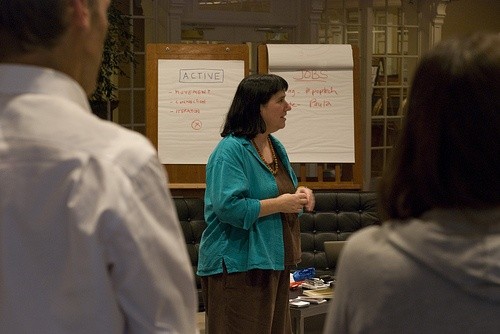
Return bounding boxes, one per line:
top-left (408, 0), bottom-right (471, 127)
top-left (303, 288), bottom-right (334, 298)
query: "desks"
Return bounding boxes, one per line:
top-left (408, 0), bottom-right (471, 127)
top-left (289, 277), bottom-right (336, 334)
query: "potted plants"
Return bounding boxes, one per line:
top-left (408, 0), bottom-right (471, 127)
top-left (88, 0), bottom-right (141, 122)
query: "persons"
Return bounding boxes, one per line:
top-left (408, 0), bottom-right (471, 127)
top-left (325, 33), bottom-right (500, 334)
top-left (196, 74), bottom-right (315, 333)
top-left (0, 0), bottom-right (198, 334)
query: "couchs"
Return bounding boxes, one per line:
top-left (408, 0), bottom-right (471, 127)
top-left (171, 192), bottom-right (384, 334)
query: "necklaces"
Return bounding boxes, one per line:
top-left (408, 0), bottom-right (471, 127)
top-left (252, 136), bottom-right (278, 174)
